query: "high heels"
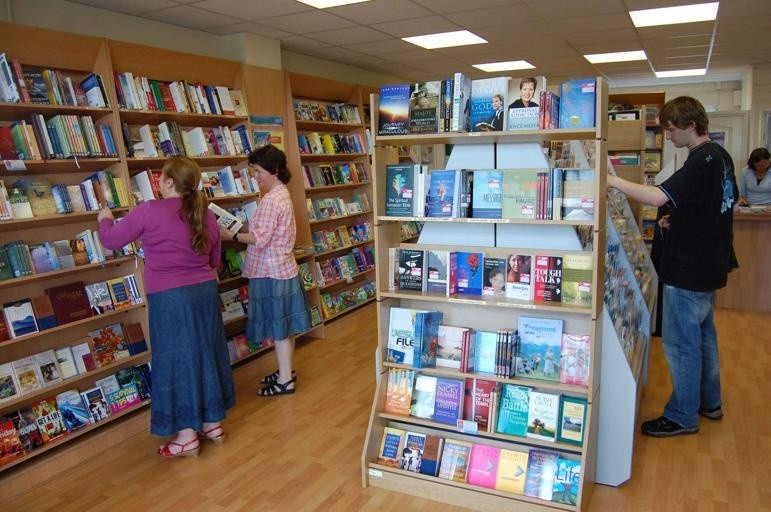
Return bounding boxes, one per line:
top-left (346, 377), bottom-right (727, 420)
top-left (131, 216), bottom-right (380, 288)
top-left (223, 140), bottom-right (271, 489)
top-left (157, 437), bottom-right (200, 458)
top-left (197, 426), bottom-right (226, 445)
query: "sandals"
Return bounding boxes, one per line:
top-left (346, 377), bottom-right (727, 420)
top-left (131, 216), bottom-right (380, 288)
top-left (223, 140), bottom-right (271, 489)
top-left (256, 380), bottom-right (295, 396)
top-left (260, 369), bottom-right (296, 384)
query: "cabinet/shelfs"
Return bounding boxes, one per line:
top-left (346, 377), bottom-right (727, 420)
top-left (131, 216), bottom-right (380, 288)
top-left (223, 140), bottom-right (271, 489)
top-left (0, 23), bottom-right (379, 479)
top-left (358, 77), bottom-right (668, 512)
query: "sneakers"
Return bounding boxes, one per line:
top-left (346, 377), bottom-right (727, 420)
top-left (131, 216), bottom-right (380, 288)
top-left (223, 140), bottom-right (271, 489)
top-left (641, 407), bottom-right (723, 438)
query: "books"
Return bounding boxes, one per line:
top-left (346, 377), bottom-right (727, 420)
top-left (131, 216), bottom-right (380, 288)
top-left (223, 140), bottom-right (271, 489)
top-left (0, 55), bottom-right (150, 470)
top-left (151, 78), bottom-right (321, 362)
top-left (293, 98), bottom-right (370, 318)
top-left (607, 103), bottom-right (657, 239)
top-left (604, 163), bottom-right (657, 370)
top-left (376, 221), bottom-right (592, 506)
top-left (370, 73), bottom-right (597, 220)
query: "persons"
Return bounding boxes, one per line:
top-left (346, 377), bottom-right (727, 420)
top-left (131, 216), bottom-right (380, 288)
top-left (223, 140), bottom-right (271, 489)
top-left (607, 95), bottom-right (739, 437)
top-left (95, 155), bottom-right (238, 460)
top-left (739, 147), bottom-right (771, 206)
top-left (218, 145), bottom-right (310, 396)
